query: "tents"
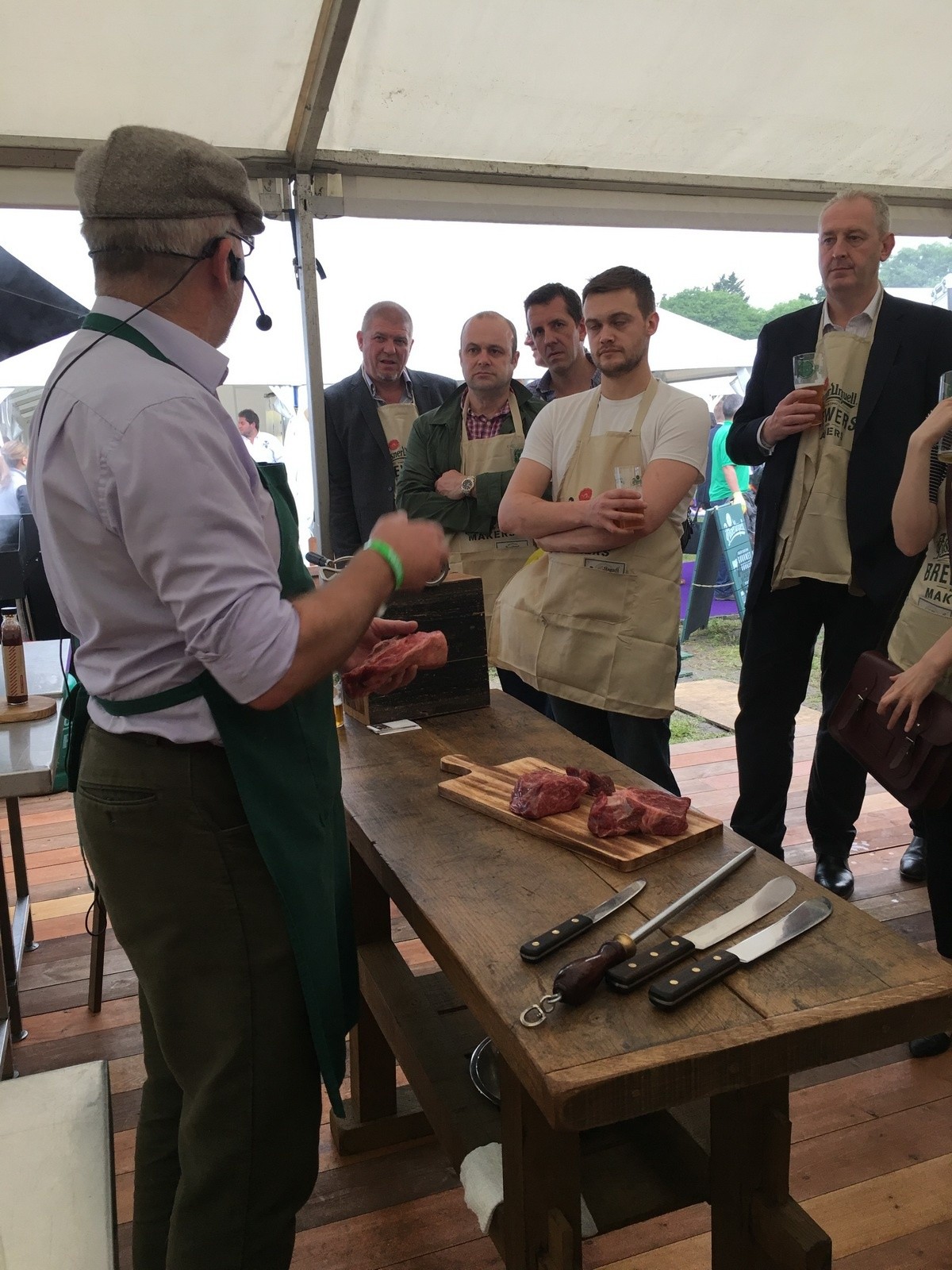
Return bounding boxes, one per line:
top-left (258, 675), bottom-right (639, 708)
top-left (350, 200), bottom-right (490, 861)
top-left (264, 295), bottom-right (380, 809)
top-left (0, 0), bottom-right (952, 518)
top-left (0, 207), bottom-right (756, 434)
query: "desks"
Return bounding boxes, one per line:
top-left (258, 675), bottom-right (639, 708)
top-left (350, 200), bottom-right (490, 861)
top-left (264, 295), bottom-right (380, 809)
top-left (0, 639), bottom-right (72, 1042)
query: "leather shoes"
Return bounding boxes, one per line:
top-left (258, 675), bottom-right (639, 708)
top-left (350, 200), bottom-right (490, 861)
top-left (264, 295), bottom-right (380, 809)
top-left (898, 834), bottom-right (929, 881)
top-left (814, 848), bottom-right (854, 900)
top-left (906, 1033), bottom-right (952, 1058)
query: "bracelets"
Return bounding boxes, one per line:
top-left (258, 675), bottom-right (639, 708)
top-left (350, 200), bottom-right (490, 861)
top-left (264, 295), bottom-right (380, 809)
top-left (363, 538), bottom-right (404, 591)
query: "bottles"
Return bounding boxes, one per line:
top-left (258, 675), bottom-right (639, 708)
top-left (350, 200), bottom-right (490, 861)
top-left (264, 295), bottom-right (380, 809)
top-left (0, 607), bottom-right (29, 705)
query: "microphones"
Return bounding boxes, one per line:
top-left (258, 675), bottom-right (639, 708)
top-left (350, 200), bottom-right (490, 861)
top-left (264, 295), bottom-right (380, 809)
top-left (229, 253), bottom-right (272, 331)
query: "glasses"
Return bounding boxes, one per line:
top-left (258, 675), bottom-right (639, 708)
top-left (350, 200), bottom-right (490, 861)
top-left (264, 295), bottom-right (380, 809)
top-left (224, 230), bottom-right (254, 255)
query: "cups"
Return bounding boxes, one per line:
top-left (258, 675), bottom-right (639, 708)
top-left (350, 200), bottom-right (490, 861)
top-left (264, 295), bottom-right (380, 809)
top-left (332, 670), bottom-right (345, 728)
top-left (614, 465), bottom-right (645, 530)
top-left (793, 352), bottom-right (825, 427)
top-left (937, 371), bottom-right (952, 465)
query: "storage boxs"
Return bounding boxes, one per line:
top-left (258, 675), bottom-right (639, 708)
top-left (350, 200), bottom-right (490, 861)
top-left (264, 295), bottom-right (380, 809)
top-left (323, 570), bottom-right (490, 726)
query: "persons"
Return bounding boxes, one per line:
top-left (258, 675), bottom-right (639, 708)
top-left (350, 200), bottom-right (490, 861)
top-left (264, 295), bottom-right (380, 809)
top-left (27, 127), bottom-right (449, 1270)
top-left (487, 267), bottom-right (710, 797)
top-left (238, 409), bottom-right (283, 464)
top-left (397, 311), bottom-right (553, 722)
top-left (524, 283), bottom-right (601, 402)
top-left (877, 398), bottom-right (952, 1056)
top-left (696, 394), bottom-right (750, 601)
top-left (0, 441), bottom-right (29, 515)
top-left (725, 189), bottom-right (952, 900)
top-left (324, 301), bottom-right (457, 571)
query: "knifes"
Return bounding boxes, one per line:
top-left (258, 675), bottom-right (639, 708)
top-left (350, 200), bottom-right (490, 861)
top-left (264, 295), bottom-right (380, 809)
top-left (648, 896), bottom-right (833, 1011)
top-left (608, 875), bottom-right (797, 994)
top-left (519, 877), bottom-right (648, 964)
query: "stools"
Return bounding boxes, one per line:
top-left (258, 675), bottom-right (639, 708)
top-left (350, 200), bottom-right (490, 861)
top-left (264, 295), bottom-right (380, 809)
top-left (0, 1059), bottom-right (119, 1270)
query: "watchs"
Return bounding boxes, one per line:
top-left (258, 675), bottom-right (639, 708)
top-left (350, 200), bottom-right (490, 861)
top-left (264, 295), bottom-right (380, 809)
top-left (461, 476), bottom-right (475, 496)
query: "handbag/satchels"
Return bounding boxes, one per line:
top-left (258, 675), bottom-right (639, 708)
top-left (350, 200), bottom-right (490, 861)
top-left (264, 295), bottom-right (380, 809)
top-left (828, 644), bottom-right (952, 813)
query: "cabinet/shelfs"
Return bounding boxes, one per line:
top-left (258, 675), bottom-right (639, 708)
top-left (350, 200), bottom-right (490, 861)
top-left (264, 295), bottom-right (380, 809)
top-left (339, 687), bottom-right (952, 1270)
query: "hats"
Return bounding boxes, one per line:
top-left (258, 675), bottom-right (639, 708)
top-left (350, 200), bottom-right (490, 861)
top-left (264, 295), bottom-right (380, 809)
top-left (74, 125), bottom-right (265, 234)
